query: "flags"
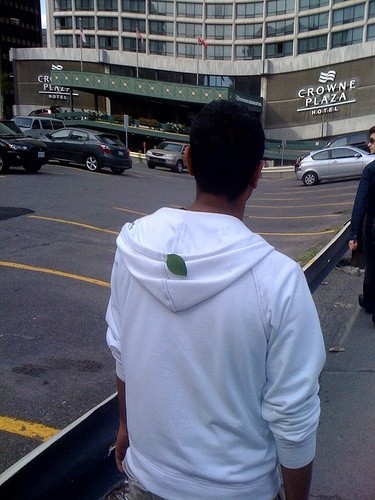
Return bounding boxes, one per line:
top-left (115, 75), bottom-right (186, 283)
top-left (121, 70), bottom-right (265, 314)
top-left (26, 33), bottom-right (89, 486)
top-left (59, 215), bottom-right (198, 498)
top-left (80, 28), bottom-right (86, 42)
top-left (137, 29), bottom-right (143, 43)
top-left (198, 35), bottom-right (208, 48)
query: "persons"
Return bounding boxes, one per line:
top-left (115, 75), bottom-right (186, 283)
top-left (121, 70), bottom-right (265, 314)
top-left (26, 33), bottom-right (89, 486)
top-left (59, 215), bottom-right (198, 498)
top-left (106, 100), bottom-right (326, 500)
top-left (348, 125), bottom-right (375, 321)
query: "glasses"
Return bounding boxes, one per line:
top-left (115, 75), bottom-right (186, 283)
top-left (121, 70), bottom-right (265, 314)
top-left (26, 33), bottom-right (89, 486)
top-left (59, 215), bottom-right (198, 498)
top-left (369, 137), bottom-right (375, 144)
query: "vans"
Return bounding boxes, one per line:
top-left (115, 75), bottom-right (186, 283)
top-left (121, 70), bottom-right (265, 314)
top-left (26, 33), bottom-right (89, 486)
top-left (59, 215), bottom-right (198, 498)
top-left (11, 116), bottom-right (68, 139)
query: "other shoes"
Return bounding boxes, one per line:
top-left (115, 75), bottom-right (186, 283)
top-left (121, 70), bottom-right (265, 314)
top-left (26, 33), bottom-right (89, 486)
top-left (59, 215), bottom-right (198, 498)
top-left (358, 293), bottom-right (374, 314)
top-left (372, 314), bottom-right (375, 325)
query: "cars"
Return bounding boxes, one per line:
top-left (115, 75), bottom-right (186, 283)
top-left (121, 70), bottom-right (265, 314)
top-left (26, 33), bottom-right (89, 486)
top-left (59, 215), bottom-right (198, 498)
top-left (0, 120), bottom-right (49, 176)
top-left (35, 126), bottom-right (133, 175)
top-left (28, 105), bottom-right (64, 116)
top-left (293, 131), bottom-right (371, 174)
top-left (146, 141), bottom-right (191, 174)
top-left (294, 147), bottom-right (375, 186)
